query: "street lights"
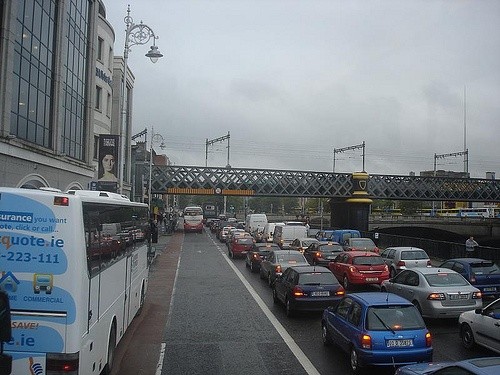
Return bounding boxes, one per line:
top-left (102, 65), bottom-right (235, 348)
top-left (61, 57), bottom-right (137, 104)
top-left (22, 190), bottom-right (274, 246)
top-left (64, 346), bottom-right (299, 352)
top-left (117, 3), bottom-right (162, 194)
top-left (148, 134), bottom-right (166, 211)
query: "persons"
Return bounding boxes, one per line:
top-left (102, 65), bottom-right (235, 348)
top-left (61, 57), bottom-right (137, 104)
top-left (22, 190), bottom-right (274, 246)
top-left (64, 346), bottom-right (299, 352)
top-left (465, 236), bottom-right (479, 257)
top-left (99, 151), bottom-right (118, 181)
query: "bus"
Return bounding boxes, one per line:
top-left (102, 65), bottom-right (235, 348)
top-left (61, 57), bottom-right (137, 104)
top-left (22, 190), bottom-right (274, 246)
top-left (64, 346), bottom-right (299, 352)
top-left (0, 186), bottom-right (159, 375)
top-left (460, 207), bottom-right (493, 221)
top-left (205, 211), bottom-right (217, 227)
top-left (182, 206), bottom-right (204, 234)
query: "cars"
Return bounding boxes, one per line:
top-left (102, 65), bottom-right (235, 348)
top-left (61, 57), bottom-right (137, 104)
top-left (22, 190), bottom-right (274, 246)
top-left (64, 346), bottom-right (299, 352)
top-left (380, 266), bottom-right (483, 327)
top-left (244, 242), bottom-right (281, 273)
top-left (290, 229), bottom-right (380, 267)
top-left (259, 250), bottom-right (310, 288)
top-left (273, 260), bottom-right (346, 318)
top-left (380, 245), bottom-right (431, 279)
top-left (432, 258), bottom-right (500, 302)
top-left (210, 215), bottom-right (256, 258)
top-left (458, 298), bottom-right (500, 355)
top-left (328, 250), bottom-right (392, 290)
top-left (394, 355), bottom-right (500, 375)
top-left (321, 283), bottom-right (434, 374)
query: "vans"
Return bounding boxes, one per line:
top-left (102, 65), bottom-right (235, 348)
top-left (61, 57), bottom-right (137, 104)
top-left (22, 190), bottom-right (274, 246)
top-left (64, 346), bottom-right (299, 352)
top-left (242, 213), bottom-right (308, 249)
